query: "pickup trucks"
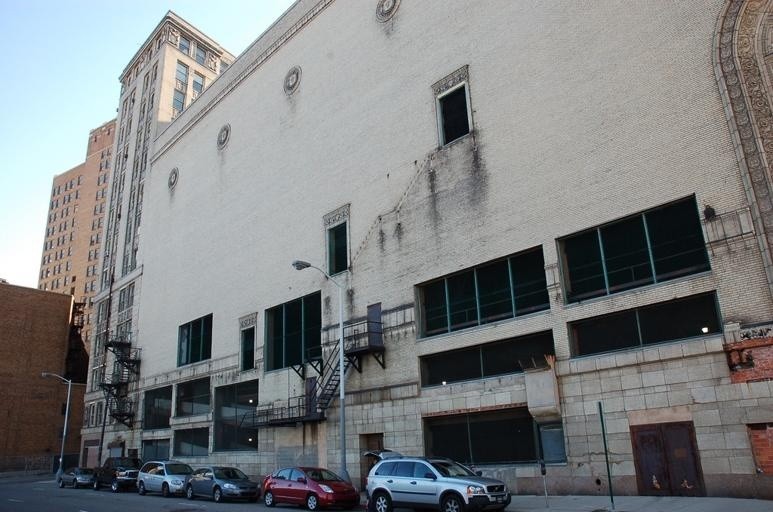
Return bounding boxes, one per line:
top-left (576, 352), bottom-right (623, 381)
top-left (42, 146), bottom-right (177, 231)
top-left (93, 456), bottom-right (143, 492)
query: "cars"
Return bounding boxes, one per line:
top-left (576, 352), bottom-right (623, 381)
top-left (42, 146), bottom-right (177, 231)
top-left (136, 459), bottom-right (193, 497)
top-left (260, 467), bottom-right (360, 510)
top-left (57, 467), bottom-right (94, 489)
top-left (183, 466), bottom-right (259, 502)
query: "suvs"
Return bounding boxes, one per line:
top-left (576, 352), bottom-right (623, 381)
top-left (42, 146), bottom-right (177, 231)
top-left (364, 449), bottom-right (511, 512)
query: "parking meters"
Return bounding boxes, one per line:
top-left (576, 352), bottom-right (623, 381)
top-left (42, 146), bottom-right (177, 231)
top-left (540, 460), bottom-right (547, 504)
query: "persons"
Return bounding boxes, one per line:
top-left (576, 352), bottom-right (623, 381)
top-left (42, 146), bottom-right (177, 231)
top-left (363, 456), bottom-right (379, 509)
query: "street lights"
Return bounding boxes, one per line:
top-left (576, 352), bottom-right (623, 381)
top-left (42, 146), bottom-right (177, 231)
top-left (293, 260), bottom-right (346, 470)
top-left (41, 373), bottom-right (71, 481)
